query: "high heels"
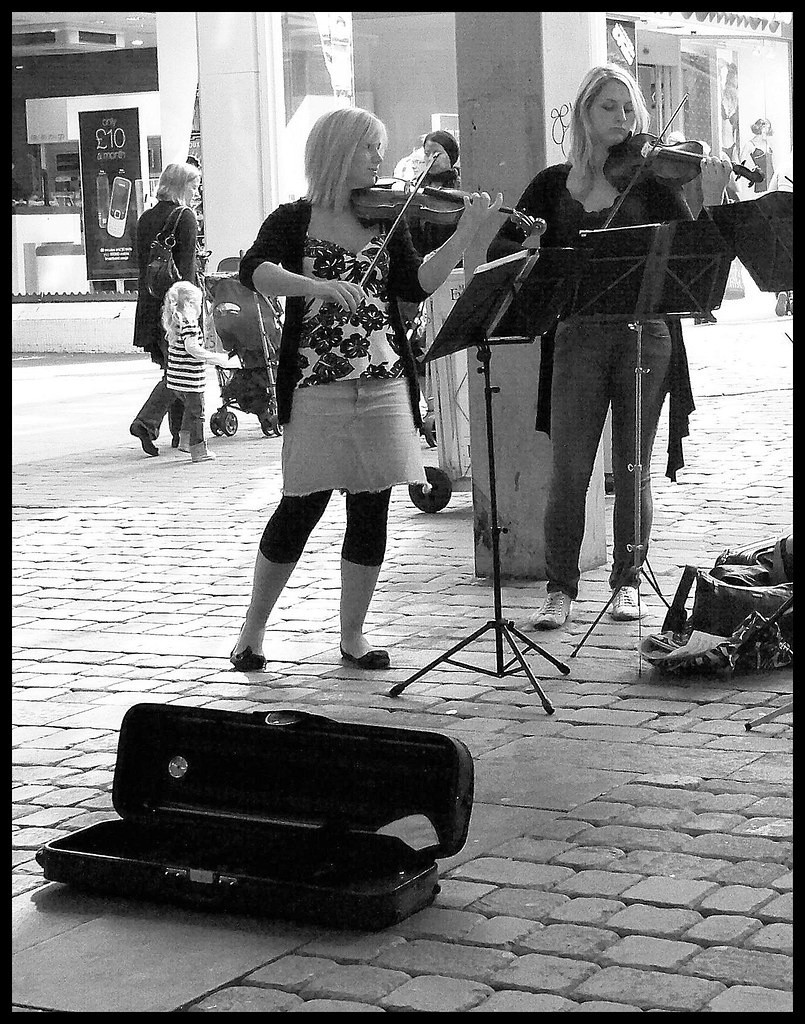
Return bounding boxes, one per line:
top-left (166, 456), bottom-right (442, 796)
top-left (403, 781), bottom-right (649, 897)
top-left (694, 310), bottom-right (717, 325)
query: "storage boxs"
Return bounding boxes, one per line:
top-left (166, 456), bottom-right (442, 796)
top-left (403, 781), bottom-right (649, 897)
top-left (34, 702), bottom-right (475, 930)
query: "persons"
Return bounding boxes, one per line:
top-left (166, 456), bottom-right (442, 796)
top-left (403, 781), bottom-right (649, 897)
top-left (395, 127), bottom-right (461, 192)
top-left (161, 281), bottom-right (227, 462)
top-left (742, 119), bottom-right (774, 193)
top-left (229, 108), bottom-right (503, 665)
top-left (130, 164), bottom-right (201, 455)
top-left (487, 66), bottom-right (733, 627)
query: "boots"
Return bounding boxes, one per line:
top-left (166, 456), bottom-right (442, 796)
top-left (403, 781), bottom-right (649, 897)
top-left (189, 439), bottom-right (216, 462)
top-left (177, 430), bottom-right (190, 453)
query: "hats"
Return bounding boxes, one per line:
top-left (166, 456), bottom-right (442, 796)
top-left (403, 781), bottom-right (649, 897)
top-left (424, 130), bottom-right (459, 168)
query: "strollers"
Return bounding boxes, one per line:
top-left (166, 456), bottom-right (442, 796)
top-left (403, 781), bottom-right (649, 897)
top-left (197, 250), bottom-right (288, 442)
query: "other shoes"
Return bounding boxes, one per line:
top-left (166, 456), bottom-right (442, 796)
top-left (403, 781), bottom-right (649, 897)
top-left (340, 641), bottom-right (390, 670)
top-left (172, 437), bottom-right (179, 448)
top-left (129, 423), bottom-right (160, 456)
top-left (775, 292), bottom-right (788, 317)
top-left (230, 620), bottom-right (267, 671)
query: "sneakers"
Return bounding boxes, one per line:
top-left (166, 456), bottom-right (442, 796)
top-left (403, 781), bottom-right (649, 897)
top-left (533, 591), bottom-right (568, 629)
top-left (611, 585), bottom-right (648, 619)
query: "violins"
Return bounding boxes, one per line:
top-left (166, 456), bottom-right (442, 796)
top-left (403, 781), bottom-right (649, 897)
top-left (602, 131), bottom-right (765, 189)
top-left (351, 175), bottom-right (547, 237)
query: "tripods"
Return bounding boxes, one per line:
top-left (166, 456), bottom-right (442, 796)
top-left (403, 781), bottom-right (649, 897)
top-left (389, 219), bottom-right (736, 719)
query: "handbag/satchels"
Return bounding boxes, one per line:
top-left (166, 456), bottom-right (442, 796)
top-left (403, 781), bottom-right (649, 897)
top-left (145, 206), bottom-right (196, 302)
top-left (640, 526), bottom-right (793, 682)
